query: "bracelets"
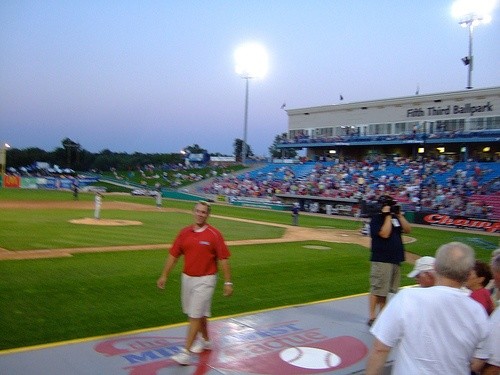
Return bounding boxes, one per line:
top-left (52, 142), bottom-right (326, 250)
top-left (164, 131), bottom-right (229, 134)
top-left (223, 282), bottom-right (233, 285)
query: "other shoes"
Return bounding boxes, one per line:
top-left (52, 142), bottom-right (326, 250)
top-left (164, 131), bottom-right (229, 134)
top-left (368, 320), bottom-right (374, 326)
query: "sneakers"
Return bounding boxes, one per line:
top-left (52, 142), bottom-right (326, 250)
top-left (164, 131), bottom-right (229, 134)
top-left (169, 347), bottom-right (192, 365)
top-left (190, 338), bottom-right (212, 353)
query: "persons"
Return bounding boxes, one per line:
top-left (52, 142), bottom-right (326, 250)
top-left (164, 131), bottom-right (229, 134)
top-left (156, 199), bottom-right (236, 366)
top-left (366, 195), bottom-right (412, 329)
top-left (6, 152), bottom-right (500, 224)
top-left (363, 243), bottom-right (500, 375)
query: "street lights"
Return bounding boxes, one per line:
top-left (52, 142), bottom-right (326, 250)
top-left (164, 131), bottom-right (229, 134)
top-left (235, 41), bottom-right (266, 165)
top-left (451, 0), bottom-right (491, 89)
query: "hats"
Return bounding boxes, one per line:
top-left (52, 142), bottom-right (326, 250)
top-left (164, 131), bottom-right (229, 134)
top-left (406, 256), bottom-right (436, 278)
